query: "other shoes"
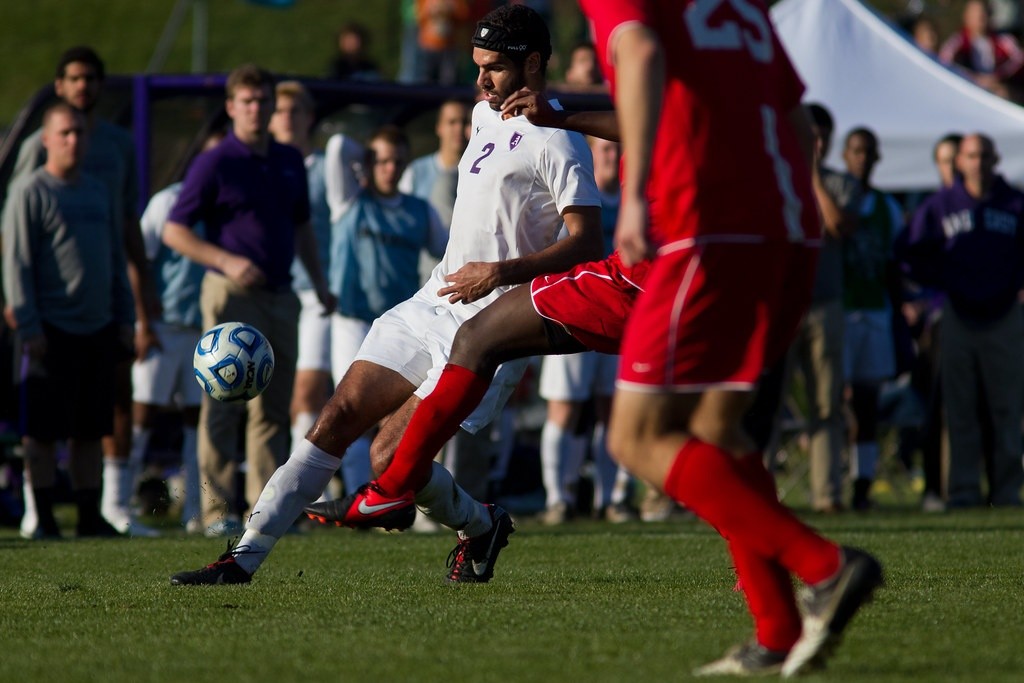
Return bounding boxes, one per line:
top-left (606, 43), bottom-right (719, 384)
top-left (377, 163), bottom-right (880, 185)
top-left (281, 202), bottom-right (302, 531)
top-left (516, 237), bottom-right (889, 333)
top-left (205, 509), bottom-right (244, 535)
top-left (812, 494), bottom-right (840, 513)
top-left (409, 509), bottom-right (442, 534)
top-left (543, 502), bottom-right (571, 525)
top-left (921, 496), bottom-right (949, 514)
top-left (608, 503), bottom-right (633, 524)
top-left (101, 506), bottom-right (153, 540)
top-left (31, 517), bottom-right (60, 536)
top-left (188, 517), bottom-right (206, 532)
top-left (642, 487), bottom-right (677, 525)
top-left (75, 510), bottom-right (121, 540)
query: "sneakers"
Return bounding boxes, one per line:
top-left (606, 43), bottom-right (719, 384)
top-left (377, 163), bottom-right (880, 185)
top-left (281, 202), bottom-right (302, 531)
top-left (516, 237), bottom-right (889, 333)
top-left (303, 482), bottom-right (416, 530)
top-left (779, 546), bottom-right (882, 677)
top-left (691, 640), bottom-right (827, 676)
top-left (168, 536), bottom-right (253, 586)
top-left (444, 502), bottom-right (516, 586)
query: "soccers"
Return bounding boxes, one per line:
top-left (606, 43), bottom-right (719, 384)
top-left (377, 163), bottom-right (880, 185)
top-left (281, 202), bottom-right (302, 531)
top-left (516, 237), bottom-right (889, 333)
top-left (192, 322), bottom-right (277, 406)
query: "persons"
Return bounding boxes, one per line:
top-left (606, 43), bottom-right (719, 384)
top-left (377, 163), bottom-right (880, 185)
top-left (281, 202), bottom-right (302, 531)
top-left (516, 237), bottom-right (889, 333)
top-left (165, 12), bottom-right (604, 590)
top-left (300, 80), bottom-right (810, 590)
top-left (1, 0), bottom-right (1024, 540)
top-left (578, 0), bottom-right (886, 681)
